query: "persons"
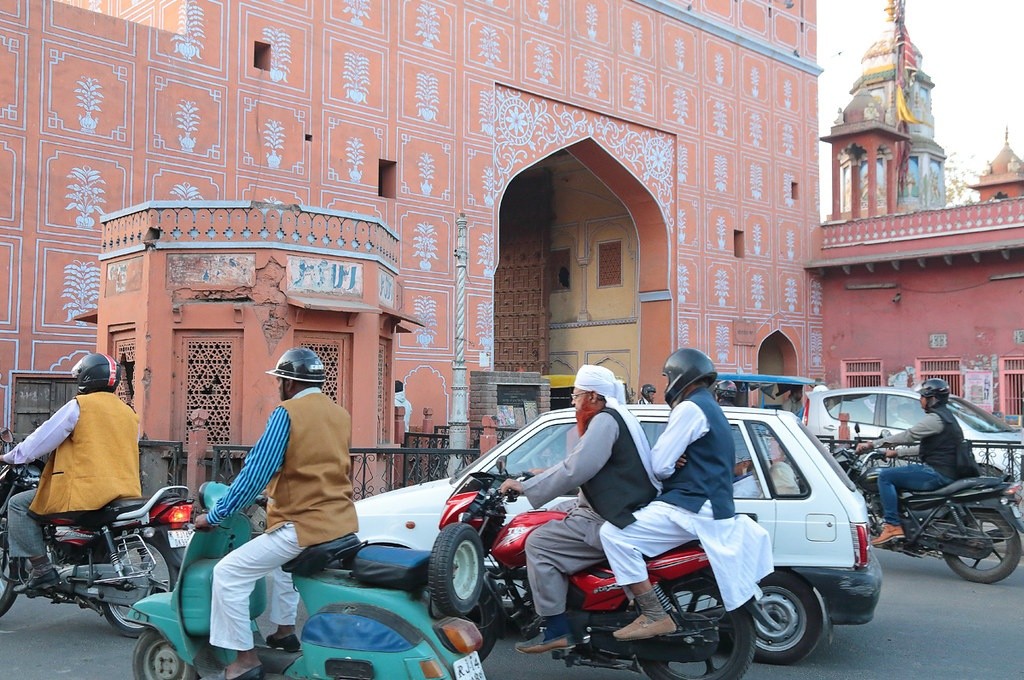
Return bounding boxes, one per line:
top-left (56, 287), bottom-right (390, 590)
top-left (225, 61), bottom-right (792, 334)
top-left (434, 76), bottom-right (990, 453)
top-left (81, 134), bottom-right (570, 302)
top-left (395, 380), bottom-right (411, 432)
top-left (599, 348), bottom-right (775, 639)
top-left (782, 385), bottom-right (828, 418)
top-left (500, 365), bottom-right (661, 654)
top-left (856, 378), bottom-right (964, 545)
top-left (0, 353), bottom-right (140, 594)
top-left (714, 380), bottom-right (737, 407)
top-left (194, 347), bottom-right (358, 680)
top-left (638, 384), bottom-right (656, 405)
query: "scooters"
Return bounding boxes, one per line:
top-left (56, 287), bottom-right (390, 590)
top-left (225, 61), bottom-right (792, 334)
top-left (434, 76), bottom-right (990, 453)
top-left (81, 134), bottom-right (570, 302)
top-left (131, 484), bottom-right (491, 680)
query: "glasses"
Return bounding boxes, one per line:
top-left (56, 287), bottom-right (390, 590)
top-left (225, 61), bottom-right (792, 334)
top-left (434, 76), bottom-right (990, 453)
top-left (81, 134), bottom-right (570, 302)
top-left (570, 392), bottom-right (591, 399)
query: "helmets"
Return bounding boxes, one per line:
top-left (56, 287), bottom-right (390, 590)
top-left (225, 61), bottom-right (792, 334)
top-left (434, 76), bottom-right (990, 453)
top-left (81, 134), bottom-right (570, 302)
top-left (714, 379), bottom-right (737, 400)
top-left (265, 348), bottom-right (327, 383)
top-left (916, 378), bottom-right (951, 411)
top-left (69, 352), bottom-right (122, 393)
top-left (660, 348), bottom-right (717, 407)
top-left (640, 384), bottom-right (656, 403)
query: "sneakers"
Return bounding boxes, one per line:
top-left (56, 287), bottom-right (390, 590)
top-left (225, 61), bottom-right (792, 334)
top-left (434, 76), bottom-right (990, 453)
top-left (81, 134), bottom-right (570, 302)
top-left (871, 525), bottom-right (905, 545)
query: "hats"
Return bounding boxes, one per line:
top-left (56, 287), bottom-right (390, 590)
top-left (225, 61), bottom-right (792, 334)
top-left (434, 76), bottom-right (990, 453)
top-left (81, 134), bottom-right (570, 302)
top-left (573, 364), bottom-right (663, 497)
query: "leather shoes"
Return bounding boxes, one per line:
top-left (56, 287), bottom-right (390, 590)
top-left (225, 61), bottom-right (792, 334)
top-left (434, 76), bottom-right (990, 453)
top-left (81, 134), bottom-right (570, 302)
top-left (265, 635), bottom-right (299, 652)
top-left (514, 632), bottom-right (576, 654)
top-left (13, 570), bottom-right (60, 594)
top-left (199, 664), bottom-right (265, 680)
top-left (612, 614), bottom-right (677, 640)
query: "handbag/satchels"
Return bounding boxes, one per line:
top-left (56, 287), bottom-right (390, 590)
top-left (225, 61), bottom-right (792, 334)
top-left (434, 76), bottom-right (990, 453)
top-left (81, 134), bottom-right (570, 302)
top-left (956, 438), bottom-right (982, 475)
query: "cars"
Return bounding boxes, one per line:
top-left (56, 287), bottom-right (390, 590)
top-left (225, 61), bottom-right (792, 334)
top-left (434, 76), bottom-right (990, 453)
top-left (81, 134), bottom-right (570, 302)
top-left (353, 402), bottom-right (883, 667)
top-left (803, 384), bottom-right (1024, 484)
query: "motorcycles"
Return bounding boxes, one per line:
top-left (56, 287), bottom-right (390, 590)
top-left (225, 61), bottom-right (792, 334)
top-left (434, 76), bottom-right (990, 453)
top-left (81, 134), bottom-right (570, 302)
top-left (0, 424), bottom-right (201, 640)
top-left (832, 420), bottom-right (1024, 585)
top-left (438, 451), bottom-right (768, 679)
